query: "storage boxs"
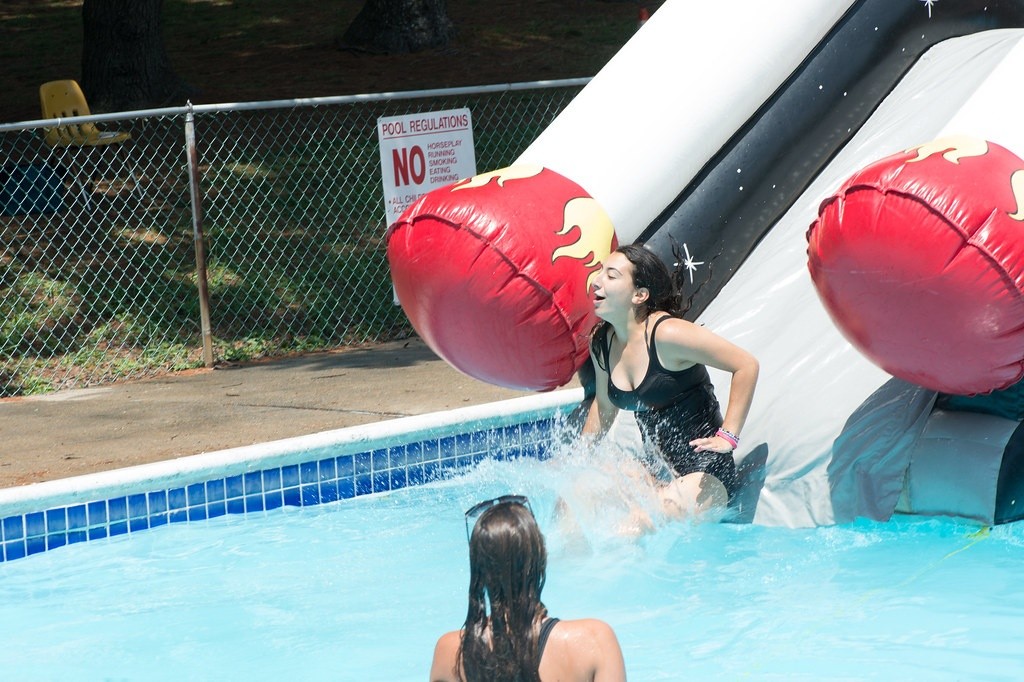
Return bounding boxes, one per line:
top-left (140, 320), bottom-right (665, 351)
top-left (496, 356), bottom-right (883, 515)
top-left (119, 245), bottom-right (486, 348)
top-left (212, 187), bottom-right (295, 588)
top-left (0, 161), bottom-right (71, 216)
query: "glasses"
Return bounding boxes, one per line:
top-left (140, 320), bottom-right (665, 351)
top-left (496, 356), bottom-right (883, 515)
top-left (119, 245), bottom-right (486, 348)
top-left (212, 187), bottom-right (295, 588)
top-left (464, 494), bottom-right (537, 546)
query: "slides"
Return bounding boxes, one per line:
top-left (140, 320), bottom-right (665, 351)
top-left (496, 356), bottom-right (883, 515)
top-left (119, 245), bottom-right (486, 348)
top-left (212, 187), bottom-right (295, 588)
top-left (379, 1), bottom-right (1024, 535)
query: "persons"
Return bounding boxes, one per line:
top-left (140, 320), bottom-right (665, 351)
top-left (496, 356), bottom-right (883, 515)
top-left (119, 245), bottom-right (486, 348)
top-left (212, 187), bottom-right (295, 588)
top-left (558, 244), bottom-right (760, 537)
top-left (430, 494), bottom-right (627, 682)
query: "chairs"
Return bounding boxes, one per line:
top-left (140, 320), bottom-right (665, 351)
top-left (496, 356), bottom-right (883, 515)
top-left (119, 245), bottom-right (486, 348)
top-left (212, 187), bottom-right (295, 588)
top-left (38, 79), bottom-right (142, 212)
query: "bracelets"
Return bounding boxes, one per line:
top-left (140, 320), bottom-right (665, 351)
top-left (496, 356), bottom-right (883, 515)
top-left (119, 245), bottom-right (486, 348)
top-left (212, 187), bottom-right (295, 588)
top-left (715, 428), bottom-right (738, 452)
